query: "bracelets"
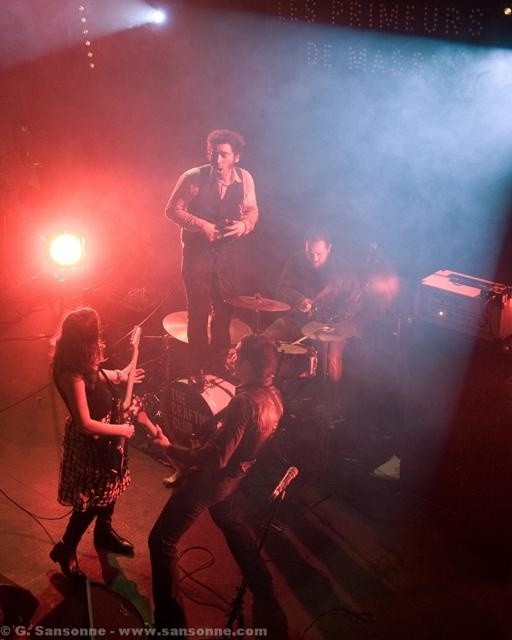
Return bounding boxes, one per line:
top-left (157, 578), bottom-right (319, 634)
top-left (114, 368), bottom-right (123, 386)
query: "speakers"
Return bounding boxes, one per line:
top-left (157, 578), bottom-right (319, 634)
top-left (27, 580), bottom-right (146, 640)
top-left (0, 573), bottom-right (39, 640)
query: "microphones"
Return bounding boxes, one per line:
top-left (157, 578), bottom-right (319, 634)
top-left (270, 464), bottom-right (300, 501)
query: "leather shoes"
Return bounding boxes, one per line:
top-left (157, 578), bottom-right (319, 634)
top-left (240, 563), bottom-right (273, 588)
top-left (49, 542), bottom-right (86, 583)
top-left (93, 528), bottom-right (135, 554)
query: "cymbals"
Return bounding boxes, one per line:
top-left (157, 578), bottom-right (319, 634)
top-left (162, 310), bottom-right (253, 347)
top-left (300, 321), bottom-right (356, 341)
top-left (222, 295), bottom-right (292, 312)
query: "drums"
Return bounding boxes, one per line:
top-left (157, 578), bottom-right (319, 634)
top-left (160, 367), bottom-right (241, 449)
top-left (274, 339), bottom-right (318, 379)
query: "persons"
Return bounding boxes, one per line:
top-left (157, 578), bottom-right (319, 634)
top-left (149, 334), bottom-right (289, 640)
top-left (260, 224), bottom-right (363, 383)
top-left (48, 306), bottom-right (145, 583)
top-left (166, 128), bottom-right (261, 390)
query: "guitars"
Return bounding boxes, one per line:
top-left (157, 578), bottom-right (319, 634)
top-left (128, 393), bottom-right (205, 489)
top-left (106, 325), bottom-right (142, 478)
